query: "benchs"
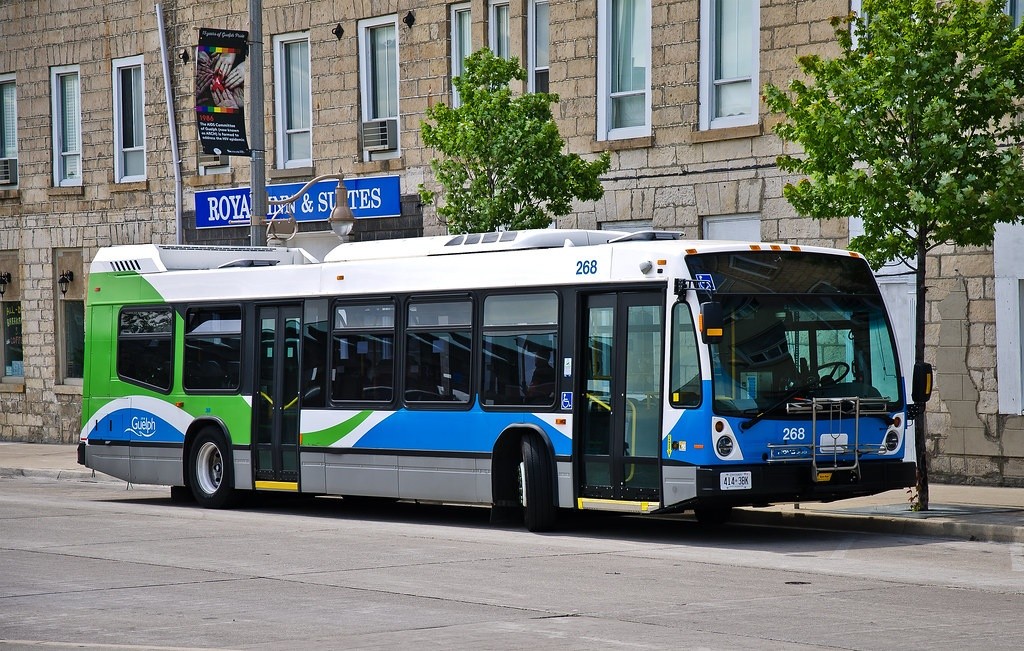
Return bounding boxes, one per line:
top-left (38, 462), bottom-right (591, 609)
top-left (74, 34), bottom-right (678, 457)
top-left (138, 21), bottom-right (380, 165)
top-left (361, 383), bottom-right (555, 406)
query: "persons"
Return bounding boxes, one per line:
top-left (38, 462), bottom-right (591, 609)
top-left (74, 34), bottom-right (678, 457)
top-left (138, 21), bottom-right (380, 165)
top-left (521, 351), bottom-right (555, 403)
top-left (752, 305), bottom-right (807, 390)
top-left (196, 52), bottom-right (247, 109)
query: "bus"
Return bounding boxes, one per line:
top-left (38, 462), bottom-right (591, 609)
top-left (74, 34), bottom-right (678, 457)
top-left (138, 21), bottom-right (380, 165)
top-left (73, 225), bottom-right (935, 535)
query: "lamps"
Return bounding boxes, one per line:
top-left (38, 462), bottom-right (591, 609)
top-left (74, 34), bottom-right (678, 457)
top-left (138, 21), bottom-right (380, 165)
top-left (0, 270), bottom-right (11, 299)
top-left (58, 269), bottom-right (73, 298)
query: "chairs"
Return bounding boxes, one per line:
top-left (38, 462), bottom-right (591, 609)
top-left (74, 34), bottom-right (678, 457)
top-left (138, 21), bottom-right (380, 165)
top-left (121, 360), bottom-right (240, 390)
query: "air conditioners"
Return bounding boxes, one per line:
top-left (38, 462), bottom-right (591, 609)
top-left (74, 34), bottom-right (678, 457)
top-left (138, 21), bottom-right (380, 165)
top-left (0, 158), bottom-right (18, 184)
top-left (361, 119), bottom-right (398, 151)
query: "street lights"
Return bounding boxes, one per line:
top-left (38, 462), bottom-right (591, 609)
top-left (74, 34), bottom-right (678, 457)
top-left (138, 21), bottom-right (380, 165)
top-left (249, 165), bottom-right (354, 251)
